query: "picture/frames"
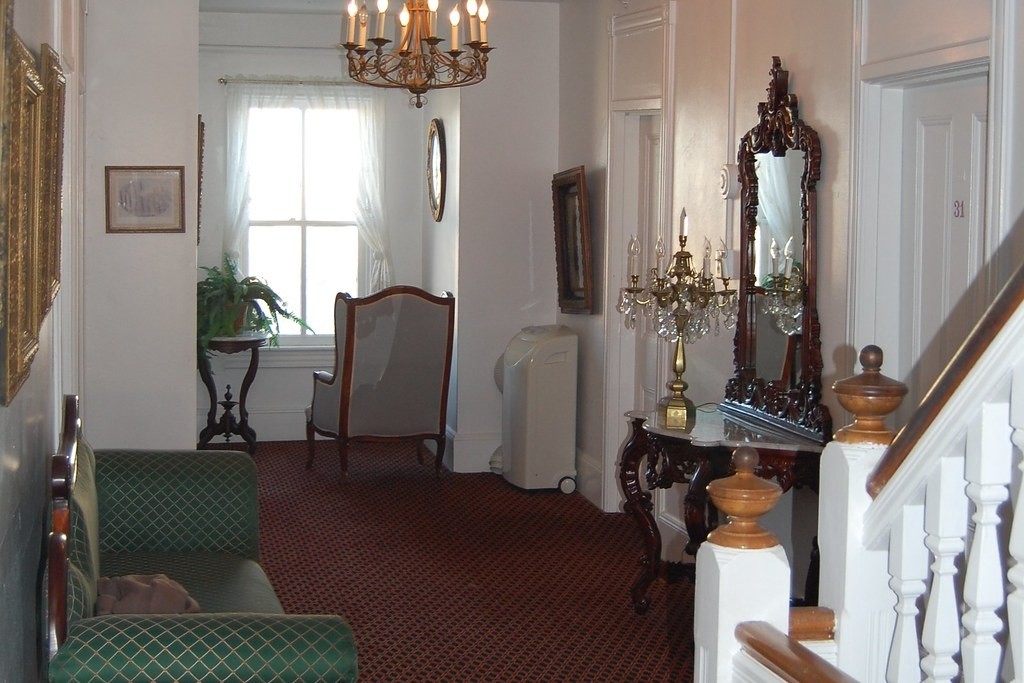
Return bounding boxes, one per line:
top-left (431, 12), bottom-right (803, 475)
top-left (551, 165), bottom-right (594, 314)
top-left (0, 0), bottom-right (67, 406)
top-left (425, 118), bottom-right (447, 223)
top-left (104, 165), bottom-right (186, 234)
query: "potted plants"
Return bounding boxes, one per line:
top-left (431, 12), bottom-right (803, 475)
top-left (196, 252), bottom-right (316, 375)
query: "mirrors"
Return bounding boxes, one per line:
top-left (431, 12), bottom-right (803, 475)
top-left (716, 55), bottom-right (835, 447)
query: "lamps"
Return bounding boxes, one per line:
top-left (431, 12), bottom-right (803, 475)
top-left (763, 236), bottom-right (802, 388)
top-left (618, 206), bottom-right (740, 431)
top-left (338, 0), bottom-right (498, 109)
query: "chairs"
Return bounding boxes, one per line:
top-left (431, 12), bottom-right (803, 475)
top-left (305, 284), bottom-right (455, 479)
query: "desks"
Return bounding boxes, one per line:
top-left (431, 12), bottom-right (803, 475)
top-left (197, 331), bottom-right (267, 457)
top-left (617, 406), bottom-right (820, 614)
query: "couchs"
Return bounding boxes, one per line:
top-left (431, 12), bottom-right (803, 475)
top-left (39, 393), bottom-right (360, 683)
